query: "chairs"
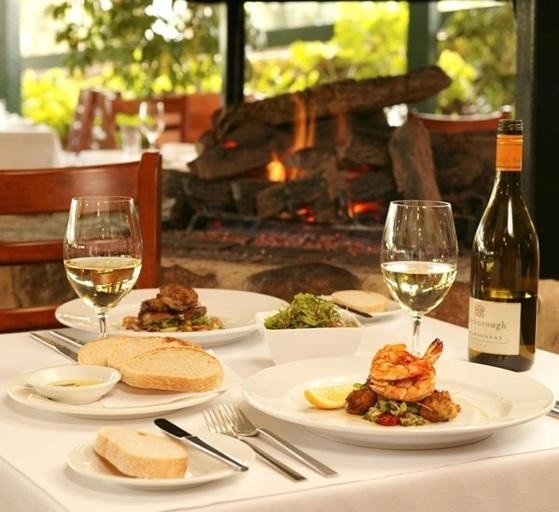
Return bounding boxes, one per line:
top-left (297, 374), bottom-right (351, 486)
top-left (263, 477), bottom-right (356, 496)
top-left (103, 91), bottom-right (190, 148)
top-left (1, 150), bottom-right (164, 333)
top-left (64, 88), bottom-right (97, 154)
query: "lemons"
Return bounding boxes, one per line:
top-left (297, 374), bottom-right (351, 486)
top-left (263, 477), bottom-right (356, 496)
top-left (305, 384), bottom-right (353, 409)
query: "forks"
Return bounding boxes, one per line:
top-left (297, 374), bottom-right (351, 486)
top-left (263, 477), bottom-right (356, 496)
top-left (217, 400), bottom-right (337, 483)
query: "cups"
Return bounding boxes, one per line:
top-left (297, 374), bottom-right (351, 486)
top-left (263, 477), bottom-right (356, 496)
top-left (119, 125), bottom-right (142, 155)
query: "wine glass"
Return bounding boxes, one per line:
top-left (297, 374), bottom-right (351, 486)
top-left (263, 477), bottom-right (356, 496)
top-left (379, 198), bottom-right (460, 360)
top-left (60, 195), bottom-right (147, 337)
top-left (138, 99), bottom-right (168, 154)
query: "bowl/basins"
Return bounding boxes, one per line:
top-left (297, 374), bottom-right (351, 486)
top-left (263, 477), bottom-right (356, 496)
top-left (28, 363), bottom-right (122, 403)
top-left (254, 308), bottom-right (366, 363)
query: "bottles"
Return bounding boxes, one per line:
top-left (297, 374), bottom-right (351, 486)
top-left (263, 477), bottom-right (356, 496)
top-left (469, 115), bottom-right (540, 373)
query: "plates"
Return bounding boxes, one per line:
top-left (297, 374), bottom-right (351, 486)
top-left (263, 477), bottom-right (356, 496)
top-left (68, 434), bottom-right (244, 490)
top-left (51, 284), bottom-right (293, 352)
top-left (241, 359), bottom-right (557, 451)
top-left (323, 290), bottom-right (407, 325)
top-left (5, 370), bottom-right (239, 422)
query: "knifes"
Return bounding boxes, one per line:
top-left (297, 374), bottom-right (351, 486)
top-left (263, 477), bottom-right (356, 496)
top-left (150, 418), bottom-right (247, 473)
top-left (319, 295), bottom-right (373, 320)
top-left (200, 407), bottom-right (305, 486)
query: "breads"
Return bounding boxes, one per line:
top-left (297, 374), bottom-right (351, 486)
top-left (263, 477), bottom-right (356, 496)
top-left (76, 336), bottom-right (224, 393)
top-left (331, 289), bottom-right (389, 314)
top-left (92, 426), bottom-right (189, 479)
